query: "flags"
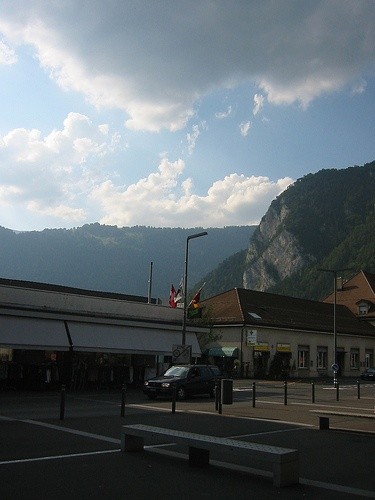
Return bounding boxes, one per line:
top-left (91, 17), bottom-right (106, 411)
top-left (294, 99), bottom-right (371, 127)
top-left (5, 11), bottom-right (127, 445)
top-left (169, 285), bottom-right (177, 308)
top-left (192, 292), bottom-right (200, 309)
top-left (174, 285), bottom-right (182, 302)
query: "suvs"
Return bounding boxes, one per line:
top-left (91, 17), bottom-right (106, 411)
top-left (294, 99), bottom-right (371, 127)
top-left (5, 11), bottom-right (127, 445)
top-left (143, 363), bottom-right (224, 402)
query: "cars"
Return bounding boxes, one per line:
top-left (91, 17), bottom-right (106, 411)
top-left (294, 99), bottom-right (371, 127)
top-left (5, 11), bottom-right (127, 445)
top-left (360, 369), bottom-right (375, 381)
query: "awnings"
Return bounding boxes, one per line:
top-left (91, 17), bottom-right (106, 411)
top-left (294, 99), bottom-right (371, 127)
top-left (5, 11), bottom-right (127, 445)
top-left (66, 321), bottom-right (202, 358)
top-left (0, 314), bottom-right (71, 351)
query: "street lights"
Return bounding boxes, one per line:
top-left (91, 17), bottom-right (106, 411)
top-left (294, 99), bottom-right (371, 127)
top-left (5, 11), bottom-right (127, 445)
top-left (316, 266), bottom-right (354, 389)
top-left (182, 231), bottom-right (209, 345)
